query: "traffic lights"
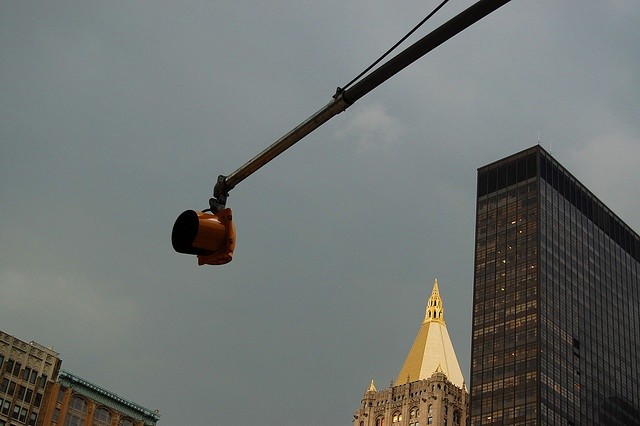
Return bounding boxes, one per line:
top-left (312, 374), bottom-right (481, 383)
top-left (172, 207), bottom-right (236, 266)
